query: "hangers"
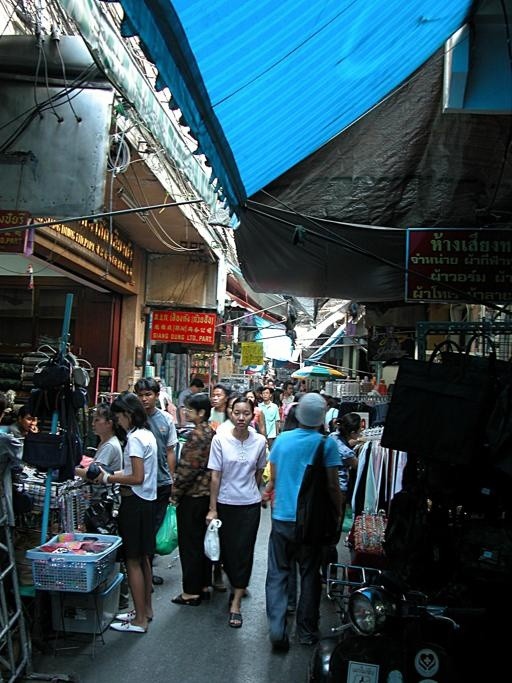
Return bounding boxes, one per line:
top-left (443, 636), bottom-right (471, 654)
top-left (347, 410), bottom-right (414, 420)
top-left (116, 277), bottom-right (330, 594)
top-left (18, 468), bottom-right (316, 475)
top-left (360, 424), bottom-right (384, 441)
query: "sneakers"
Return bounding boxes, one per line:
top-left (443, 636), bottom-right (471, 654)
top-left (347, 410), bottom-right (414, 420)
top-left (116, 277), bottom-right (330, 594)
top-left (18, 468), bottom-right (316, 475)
top-left (270, 634), bottom-right (290, 654)
top-left (295, 628), bottom-right (315, 646)
top-left (149, 574), bottom-right (164, 594)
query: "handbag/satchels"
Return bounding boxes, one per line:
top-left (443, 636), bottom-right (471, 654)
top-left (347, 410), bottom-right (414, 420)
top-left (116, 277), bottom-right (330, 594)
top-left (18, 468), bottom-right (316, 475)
top-left (87, 461), bottom-right (118, 482)
top-left (295, 434), bottom-right (347, 550)
top-left (61, 430), bottom-right (86, 483)
top-left (22, 432), bottom-right (64, 468)
top-left (204, 518), bottom-right (224, 563)
top-left (27, 357), bottom-right (93, 420)
top-left (381, 334), bottom-right (512, 468)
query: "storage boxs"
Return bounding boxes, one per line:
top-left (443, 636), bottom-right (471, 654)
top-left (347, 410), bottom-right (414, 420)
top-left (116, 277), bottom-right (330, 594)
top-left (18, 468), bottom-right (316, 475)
top-left (48, 572), bottom-right (126, 636)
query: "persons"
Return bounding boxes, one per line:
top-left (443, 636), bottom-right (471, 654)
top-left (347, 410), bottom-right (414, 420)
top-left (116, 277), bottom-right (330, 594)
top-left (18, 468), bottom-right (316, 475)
top-left (265, 393), bottom-right (344, 652)
top-left (10, 404), bottom-right (39, 460)
top-left (74, 376), bottom-right (387, 633)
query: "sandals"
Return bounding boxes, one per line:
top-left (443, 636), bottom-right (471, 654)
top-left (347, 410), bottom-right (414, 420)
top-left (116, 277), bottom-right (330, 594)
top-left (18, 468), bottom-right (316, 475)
top-left (171, 587), bottom-right (211, 606)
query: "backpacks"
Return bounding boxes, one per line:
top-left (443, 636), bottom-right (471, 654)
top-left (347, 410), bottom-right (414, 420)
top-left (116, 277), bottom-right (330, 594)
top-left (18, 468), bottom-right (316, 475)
top-left (84, 499), bottom-right (124, 561)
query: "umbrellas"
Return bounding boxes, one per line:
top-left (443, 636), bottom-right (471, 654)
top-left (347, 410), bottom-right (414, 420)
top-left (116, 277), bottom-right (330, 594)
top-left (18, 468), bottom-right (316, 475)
top-left (290, 363), bottom-right (343, 390)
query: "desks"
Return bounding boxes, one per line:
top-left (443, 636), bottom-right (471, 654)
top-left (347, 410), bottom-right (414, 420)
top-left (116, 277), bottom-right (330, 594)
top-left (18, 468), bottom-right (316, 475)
top-left (33, 559), bottom-right (122, 662)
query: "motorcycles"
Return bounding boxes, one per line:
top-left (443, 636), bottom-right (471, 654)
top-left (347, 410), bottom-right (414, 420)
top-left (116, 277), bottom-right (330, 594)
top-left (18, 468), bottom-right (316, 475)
top-left (310, 557), bottom-right (483, 683)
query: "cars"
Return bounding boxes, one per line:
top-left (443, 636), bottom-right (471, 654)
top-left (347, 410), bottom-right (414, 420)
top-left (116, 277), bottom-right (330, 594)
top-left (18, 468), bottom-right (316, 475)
top-left (215, 376), bottom-right (261, 392)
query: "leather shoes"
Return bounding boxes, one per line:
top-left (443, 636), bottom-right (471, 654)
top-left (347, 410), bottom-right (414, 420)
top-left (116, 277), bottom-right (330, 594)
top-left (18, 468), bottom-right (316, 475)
top-left (109, 608), bottom-right (153, 634)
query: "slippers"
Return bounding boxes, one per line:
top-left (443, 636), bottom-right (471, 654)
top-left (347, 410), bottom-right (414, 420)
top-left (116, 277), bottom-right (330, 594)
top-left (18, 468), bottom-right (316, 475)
top-left (229, 605), bottom-right (243, 628)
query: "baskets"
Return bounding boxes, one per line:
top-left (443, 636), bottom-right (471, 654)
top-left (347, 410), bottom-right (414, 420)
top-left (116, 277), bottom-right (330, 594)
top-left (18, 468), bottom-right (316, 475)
top-left (25, 531), bottom-right (124, 593)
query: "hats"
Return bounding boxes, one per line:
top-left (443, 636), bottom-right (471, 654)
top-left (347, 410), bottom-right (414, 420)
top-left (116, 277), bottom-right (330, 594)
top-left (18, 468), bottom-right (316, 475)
top-left (295, 392), bottom-right (327, 427)
